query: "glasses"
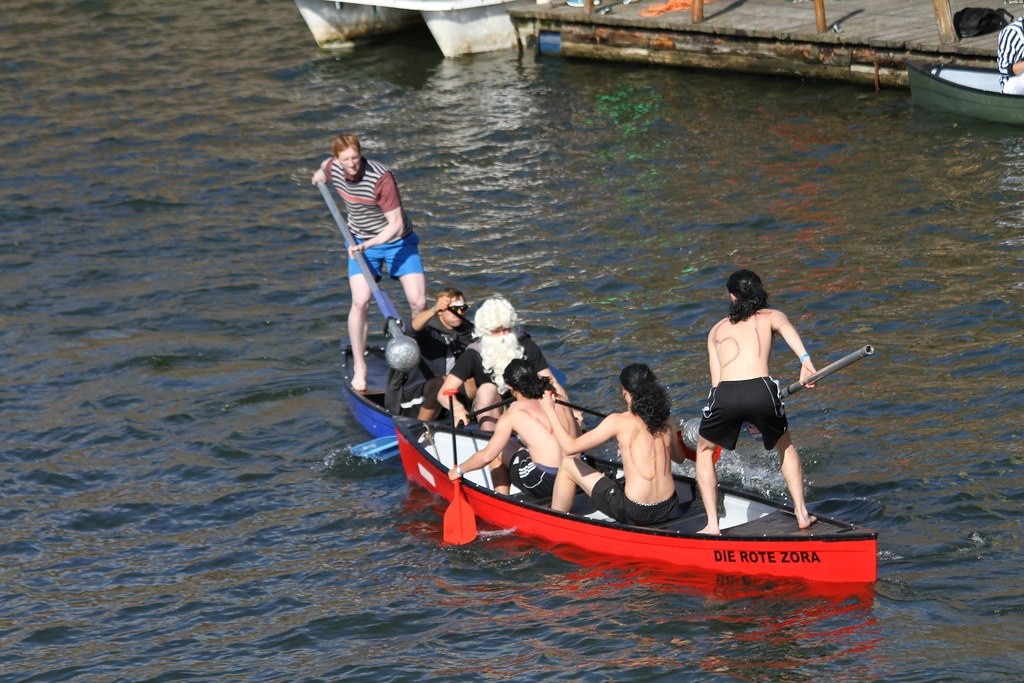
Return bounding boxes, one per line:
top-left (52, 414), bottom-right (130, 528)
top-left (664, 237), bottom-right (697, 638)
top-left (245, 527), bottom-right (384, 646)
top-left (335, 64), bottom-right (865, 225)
top-left (449, 304), bottom-right (468, 312)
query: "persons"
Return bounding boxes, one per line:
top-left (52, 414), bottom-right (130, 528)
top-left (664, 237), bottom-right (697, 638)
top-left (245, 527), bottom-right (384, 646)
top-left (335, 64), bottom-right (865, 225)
top-left (312, 133), bottom-right (427, 392)
top-left (997, 11), bottom-right (1024, 96)
top-left (447, 358), bottom-right (585, 499)
top-left (696, 269), bottom-right (817, 536)
top-left (435, 296), bottom-right (572, 438)
top-left (536, 363), bottom-right (685, 528)
top-left (385, 286), bottom-right (476, 423)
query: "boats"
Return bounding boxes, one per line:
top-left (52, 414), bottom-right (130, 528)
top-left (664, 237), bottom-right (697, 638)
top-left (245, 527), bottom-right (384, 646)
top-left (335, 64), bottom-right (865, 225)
top-left (392, 414), bottom-right (878, 595)
top-left (905, 60), bottom-right (1024, 125)
top-left (340, 337), bottom-right (522, 449)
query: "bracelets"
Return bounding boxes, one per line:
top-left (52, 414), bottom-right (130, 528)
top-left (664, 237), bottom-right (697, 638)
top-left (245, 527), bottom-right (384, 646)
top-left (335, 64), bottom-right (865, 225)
top-left (453, 403), bottom-right (463, 409)
top-left (457, 466), bottom-right (463, 475)
top-left (359, 243), bottom-right (364, 251)
top-left (800, 353), bottom-right (810, 363)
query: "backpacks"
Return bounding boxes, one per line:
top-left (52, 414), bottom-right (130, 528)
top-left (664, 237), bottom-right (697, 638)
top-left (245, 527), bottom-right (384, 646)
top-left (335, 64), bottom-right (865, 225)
top-left (953, 6), bottom-right (1014, 38)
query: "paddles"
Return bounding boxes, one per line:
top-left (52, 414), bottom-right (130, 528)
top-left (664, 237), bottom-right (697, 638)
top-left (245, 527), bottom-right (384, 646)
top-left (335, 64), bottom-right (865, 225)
top-left (440, 389), bottom-right (479, 546)
top-left (546, 390), bottom-right (726, 465)
top-left (349, 396), bottom-right (517, 462)
